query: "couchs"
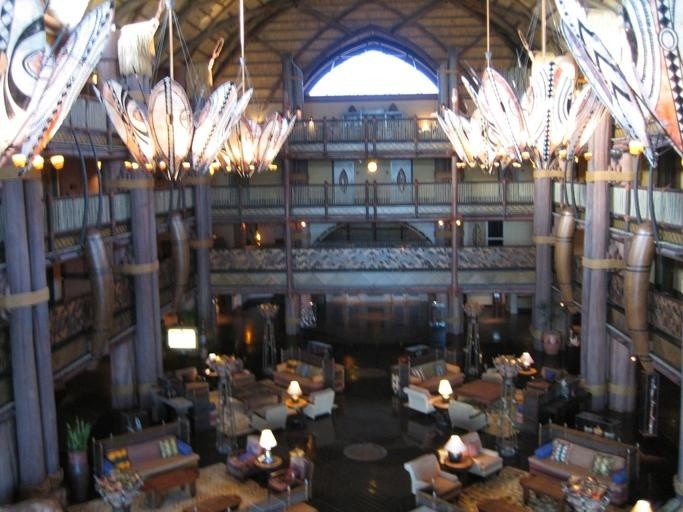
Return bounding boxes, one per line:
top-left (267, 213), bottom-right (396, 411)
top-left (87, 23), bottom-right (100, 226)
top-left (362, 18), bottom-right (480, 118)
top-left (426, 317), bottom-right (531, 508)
top-left (89, 347), bottom-right (344, 512)
top-left (388, 346), bottom-right (651, 512)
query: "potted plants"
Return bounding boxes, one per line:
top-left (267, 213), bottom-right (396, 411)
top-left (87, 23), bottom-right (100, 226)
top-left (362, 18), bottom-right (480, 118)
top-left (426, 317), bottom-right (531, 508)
top-left (60, 415), bottom-right (93, 501)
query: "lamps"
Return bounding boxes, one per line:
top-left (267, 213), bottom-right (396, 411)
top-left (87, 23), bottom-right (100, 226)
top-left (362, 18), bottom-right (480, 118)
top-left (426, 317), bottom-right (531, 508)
top-left (0, 2), bottom-right (116, 178)
top-left (206, 1), bottom-right (296, 178)
top-left (434, 1), bottom-right (527, 176)
top-left (548, 0), bottom-right (681, 174)
top-left (88, 0), bottom-right (255, 181)
top-left (462, 0), bottom-right (603, 172)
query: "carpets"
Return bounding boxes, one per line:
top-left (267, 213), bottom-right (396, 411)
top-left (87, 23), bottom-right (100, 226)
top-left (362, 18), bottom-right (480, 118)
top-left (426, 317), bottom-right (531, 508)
top-left (63, 461), bottom-right (319, 512)
